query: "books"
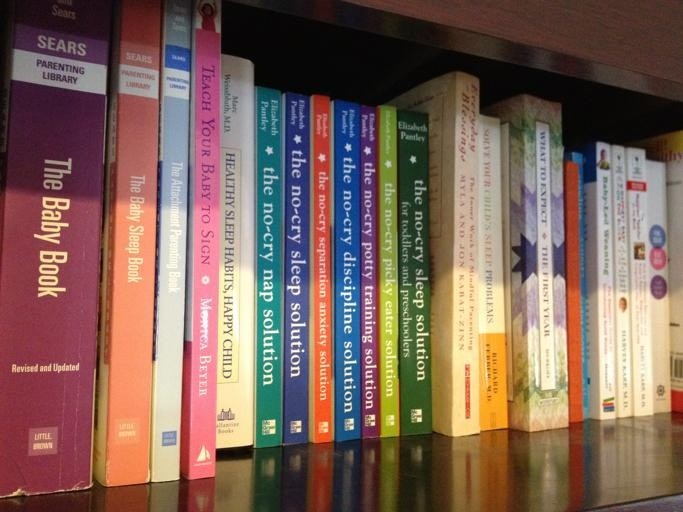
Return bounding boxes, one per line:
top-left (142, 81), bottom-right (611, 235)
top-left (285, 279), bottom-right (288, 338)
top-left (2, 2), bottom-right (681, 501)
top-left (2, 411), bottom-right (676, 512)
